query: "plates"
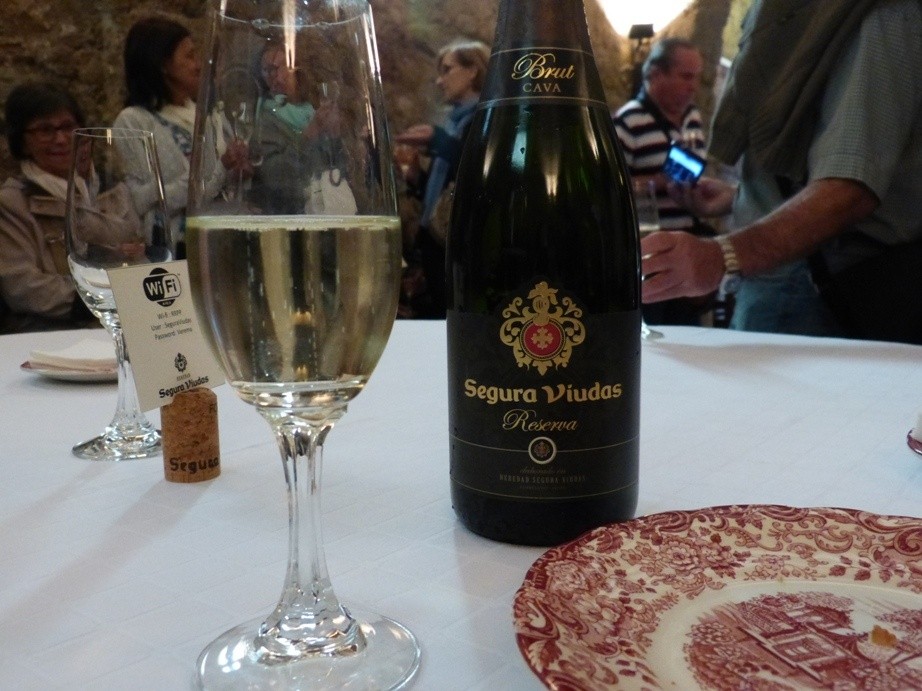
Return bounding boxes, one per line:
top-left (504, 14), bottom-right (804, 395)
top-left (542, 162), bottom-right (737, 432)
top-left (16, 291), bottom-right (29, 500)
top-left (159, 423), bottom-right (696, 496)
top-left (510, 503), bottom-right (922, 691)
top-left (17, 359), bottom-right (118, 383)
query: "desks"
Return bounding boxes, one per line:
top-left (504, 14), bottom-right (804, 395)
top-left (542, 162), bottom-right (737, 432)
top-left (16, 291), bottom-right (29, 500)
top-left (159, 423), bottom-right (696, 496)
top-left (1, 320), bottom-right (922, 690)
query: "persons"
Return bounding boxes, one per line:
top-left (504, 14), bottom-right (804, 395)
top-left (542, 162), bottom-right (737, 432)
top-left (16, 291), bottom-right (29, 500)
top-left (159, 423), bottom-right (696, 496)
top-left (1, 78), bottom-right (145, 334)
top-left (245, 39), bottom-right (356, 215)
top-left (393, 39), bottom-right (492, 319)
top-left (640, 1), bottom-right (921, 345)
top-left (109, 14), bottom-right (250, 262)
top-left (611, 38), bottom-right (708, 240)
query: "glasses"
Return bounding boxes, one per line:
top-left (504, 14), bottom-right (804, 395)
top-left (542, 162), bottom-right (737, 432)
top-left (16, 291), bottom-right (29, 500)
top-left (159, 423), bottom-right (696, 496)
top-left (438, 64), bottom-right (461, 76)
top-left (23, 119), bottom-right (78, 142)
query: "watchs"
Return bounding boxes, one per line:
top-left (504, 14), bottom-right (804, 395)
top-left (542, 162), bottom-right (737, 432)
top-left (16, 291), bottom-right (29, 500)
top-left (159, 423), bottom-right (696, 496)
top-left (713, 233), bottom-right (747, 295)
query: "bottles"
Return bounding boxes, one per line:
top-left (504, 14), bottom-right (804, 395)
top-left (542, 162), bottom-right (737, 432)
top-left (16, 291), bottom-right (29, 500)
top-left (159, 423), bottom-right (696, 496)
top-left (447, 0), bottom-right (641, 544)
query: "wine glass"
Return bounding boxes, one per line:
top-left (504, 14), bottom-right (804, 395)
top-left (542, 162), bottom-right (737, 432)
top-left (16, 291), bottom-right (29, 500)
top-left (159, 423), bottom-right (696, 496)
top-left (188, 0), bottom-right (424, 691)
top-left (629, 177), bottom-right (663, 341)
top-left (65, 127), bottom-right (174, 461)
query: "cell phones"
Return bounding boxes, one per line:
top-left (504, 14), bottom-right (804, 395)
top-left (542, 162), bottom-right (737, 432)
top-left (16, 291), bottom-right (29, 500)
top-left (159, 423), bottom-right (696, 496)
top-left (662, 140), bottom-right (707, 189)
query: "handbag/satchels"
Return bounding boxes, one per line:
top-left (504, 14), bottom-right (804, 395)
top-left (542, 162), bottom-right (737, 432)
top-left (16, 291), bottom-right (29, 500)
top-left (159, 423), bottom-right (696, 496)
top-left (304, 169), bottom-right (359, 217)
top-left (431, 181), bottom-right (456, 240)
